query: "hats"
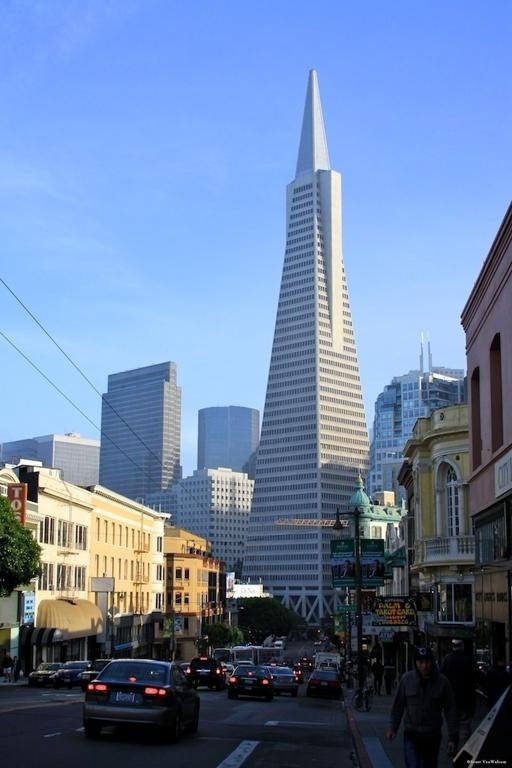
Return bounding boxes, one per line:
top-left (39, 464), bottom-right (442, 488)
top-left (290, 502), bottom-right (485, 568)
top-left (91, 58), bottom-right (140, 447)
top-left (414, 646), bottom-right (434, 661)
top-left (450, 639), bottom-right (464, 648)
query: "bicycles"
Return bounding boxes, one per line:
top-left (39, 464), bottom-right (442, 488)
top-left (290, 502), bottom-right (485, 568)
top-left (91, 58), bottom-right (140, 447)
top-left (350, 680), bottom-right (376, 712)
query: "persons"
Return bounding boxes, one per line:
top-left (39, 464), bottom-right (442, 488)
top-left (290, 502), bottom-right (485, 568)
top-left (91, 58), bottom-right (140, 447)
top-left (371, 657), bottom-right (385, 695)
top-left (12, 655), bottom-right (23, 682)
top-left (443, 638), bottom-right (479, 754)
top-left (1, 651), bottom-right (13, 682)
top-left (384, 649), bottom-right (457, 766)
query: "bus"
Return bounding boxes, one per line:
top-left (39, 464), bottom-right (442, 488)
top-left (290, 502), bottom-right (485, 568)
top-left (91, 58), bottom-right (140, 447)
top-left (211, 636), bottom-right (287, 666)
top-left (312, 651), bottom-right (344, 669)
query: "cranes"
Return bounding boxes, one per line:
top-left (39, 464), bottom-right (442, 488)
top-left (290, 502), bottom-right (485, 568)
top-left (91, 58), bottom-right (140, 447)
top-left (272, 517), bottom-right (350, 528)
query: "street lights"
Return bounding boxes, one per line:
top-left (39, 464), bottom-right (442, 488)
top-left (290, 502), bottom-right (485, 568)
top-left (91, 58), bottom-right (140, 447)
top-left (334, 506), bottom-right (364, 701)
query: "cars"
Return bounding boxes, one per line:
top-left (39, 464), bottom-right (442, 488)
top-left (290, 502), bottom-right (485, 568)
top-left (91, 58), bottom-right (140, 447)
top-left (226, 656), bottom-right (342, 702)
top-left (26, 654), bottom-right (225, 744)
